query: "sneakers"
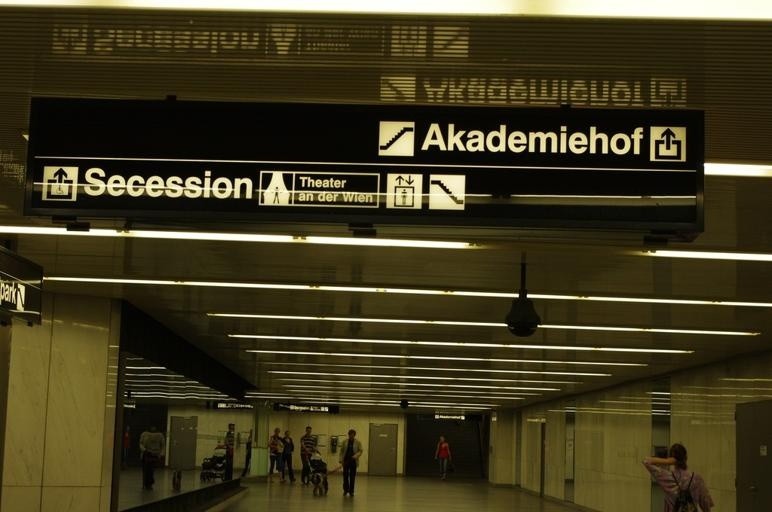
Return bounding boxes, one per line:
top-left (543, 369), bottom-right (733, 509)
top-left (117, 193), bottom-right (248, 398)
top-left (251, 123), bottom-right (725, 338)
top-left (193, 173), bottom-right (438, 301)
top-left (280, 478), bottom-right (296, 482)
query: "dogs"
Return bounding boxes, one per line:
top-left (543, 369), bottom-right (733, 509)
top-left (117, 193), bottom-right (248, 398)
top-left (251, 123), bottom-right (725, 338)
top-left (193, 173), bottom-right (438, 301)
top-left (171, 466), bottom-right (182, 492)
top-left (311, 471), bottom-right (330, 497)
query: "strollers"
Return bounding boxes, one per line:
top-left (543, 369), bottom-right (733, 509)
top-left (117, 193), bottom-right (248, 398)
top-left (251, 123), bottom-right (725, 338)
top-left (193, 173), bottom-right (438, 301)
top-left (304, 449), bottom-right (327, 484)
top-left (201, 453), bottom-right (226, 481)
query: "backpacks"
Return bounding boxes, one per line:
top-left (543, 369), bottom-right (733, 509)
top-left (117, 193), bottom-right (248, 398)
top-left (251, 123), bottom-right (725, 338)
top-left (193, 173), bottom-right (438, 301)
top-left (671, 471), bottom-right (698, 511)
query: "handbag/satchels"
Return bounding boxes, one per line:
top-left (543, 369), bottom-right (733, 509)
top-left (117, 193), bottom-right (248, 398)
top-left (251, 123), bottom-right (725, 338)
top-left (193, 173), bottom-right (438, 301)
top-left (277, 441), bottom-right (284, 452)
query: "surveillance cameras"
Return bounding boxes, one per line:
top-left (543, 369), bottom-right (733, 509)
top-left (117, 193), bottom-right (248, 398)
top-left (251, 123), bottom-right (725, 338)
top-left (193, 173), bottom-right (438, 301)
top-left (505, 251), bottom-right (541, 337)
top-left (399, 400), bottom-right (410, 409)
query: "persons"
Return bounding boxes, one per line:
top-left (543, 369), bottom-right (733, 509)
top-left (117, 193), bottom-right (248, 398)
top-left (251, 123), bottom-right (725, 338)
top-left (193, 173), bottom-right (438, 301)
top-left (268, 426), bottom-right (288, 482)
top-left (300, 425), bottom-right (322, 485)
top-left (241, 428), bottom-right (251, 476)
top-left (282, 430), bottom-right (296, 482)
top-left (435, 433), bottom-right (452, 480)
top-left (337, 428), bottom-right (364, 496)
top-left (641, 442), bottom-right (714, 512)
top-left (225, 423), bottom-right (235, 481)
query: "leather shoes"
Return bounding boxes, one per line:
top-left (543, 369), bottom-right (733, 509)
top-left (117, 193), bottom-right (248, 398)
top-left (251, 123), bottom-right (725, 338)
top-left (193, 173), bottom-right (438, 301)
top-left (342, 491), bottom-right (354, 496)
